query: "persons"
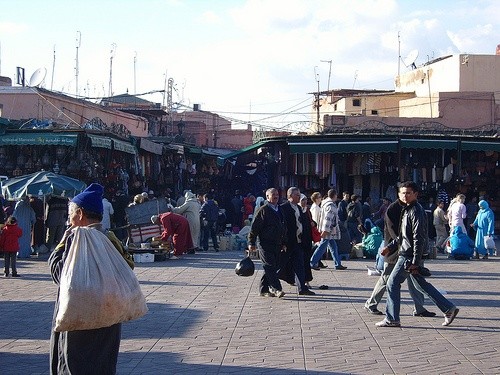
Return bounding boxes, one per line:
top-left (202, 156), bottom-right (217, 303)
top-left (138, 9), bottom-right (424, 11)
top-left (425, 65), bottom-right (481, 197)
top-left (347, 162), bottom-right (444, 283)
top-left (0, 173), bottom-right (159, 259)
top-left (289, 193), bottom-right (318, 289)
top-left (375, 181), bottom-right (459, 327)
top-left (418, 192), bottom-right (495, 259)
top-left (0, 216), bottom-right (23, 278)
top-left (309, 188), bottom-right (398, 270)
top-left (247, 187), bottom-right (289, 298)
top-left (151, 212), bottom-right (194, 260)
top-left (155, 184), bottom-right (288, 253)
top-left (269, 186), bottom-right (315, 295)
top-left (47, 183), bottom-right (135, 375)
top-left (364, 180), bottom-right (436, 317)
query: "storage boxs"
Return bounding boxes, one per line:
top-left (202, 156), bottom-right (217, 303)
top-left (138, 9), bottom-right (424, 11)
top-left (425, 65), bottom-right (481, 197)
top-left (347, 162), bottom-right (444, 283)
top-left (141, 243), bottom-right (151, 248)
top-left (134, 253), bottom-right (154, 262)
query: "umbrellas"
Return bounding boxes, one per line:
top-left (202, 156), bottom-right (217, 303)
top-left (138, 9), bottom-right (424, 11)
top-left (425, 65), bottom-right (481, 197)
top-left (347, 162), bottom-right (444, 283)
top-left (1, 168), bottom-right (87, 224)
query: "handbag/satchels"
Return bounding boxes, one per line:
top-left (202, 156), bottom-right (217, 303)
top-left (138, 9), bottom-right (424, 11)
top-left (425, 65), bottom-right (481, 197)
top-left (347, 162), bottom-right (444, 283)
top-left (484, 235), bottom-right (495, 249)
top-left (234, 252), bottom-right (254, 277)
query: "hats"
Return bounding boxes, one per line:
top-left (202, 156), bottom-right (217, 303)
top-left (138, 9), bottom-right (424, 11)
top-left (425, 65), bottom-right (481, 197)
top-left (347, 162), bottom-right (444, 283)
top-left (71, 183), bottom-right (104, 213)
top-left (151, 216), bottom-right (157, 223)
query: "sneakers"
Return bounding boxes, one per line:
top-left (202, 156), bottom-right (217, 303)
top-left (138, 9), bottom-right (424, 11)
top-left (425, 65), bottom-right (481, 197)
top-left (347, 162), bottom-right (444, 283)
top-left (442, 306), bottom-right (459, 326)
top-left (413, 310), bottom-right (436, 317)
top-left (378, 320), bottom-right (400, 327)
top-left (365, 308), bottom-right (382, 315)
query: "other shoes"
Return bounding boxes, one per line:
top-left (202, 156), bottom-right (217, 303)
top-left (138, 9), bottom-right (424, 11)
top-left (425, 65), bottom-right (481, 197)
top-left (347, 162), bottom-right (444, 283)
top-left (433, 245), bottom-right (444, 254)
top-left (311, 266), bottom-right (320, 270)
top-left (12, 274), bottom-right (20, 277)
top-left (170, 256), bottom-right (179, 259)
top-left (187, 250), bottom-right (195, 254)
top-left (319, 263), bottom-right (328, 268)
top-left (299, 290), bottom-right (314, 295)
top-left (482, 256), bottom-right (488, 260)
top-left (470, 257), bottom-right (479, 260)
top-left (276, 290), bottom-right (284, 298)
top-left (203, 249), bottom-right (207, 252)
top-left (4, 273), bottom-right (8, 277)
top-left (337, 266), bottom-right (347, 270)
top-left (216, 248), bottom-right (219, 252)
top-left (261, 291), bottom-right (274, 297)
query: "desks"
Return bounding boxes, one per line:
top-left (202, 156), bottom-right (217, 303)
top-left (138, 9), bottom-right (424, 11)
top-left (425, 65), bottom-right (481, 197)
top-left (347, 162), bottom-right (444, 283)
top-left (127, 248), bottom-right (170, 260)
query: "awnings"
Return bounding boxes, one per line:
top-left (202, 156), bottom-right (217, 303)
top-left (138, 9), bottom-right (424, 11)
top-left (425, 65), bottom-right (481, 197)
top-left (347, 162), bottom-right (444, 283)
top-left (0, 130), bottom-right (238, 156)
top-left (286, 136), bottom-right (500, 155)
top-left (216, 138), bottom-right (286, 166)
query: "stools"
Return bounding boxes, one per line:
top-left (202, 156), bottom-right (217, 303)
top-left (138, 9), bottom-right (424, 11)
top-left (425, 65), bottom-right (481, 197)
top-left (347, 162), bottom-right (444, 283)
top-left (339, 254), bottom-right (350, 260)
